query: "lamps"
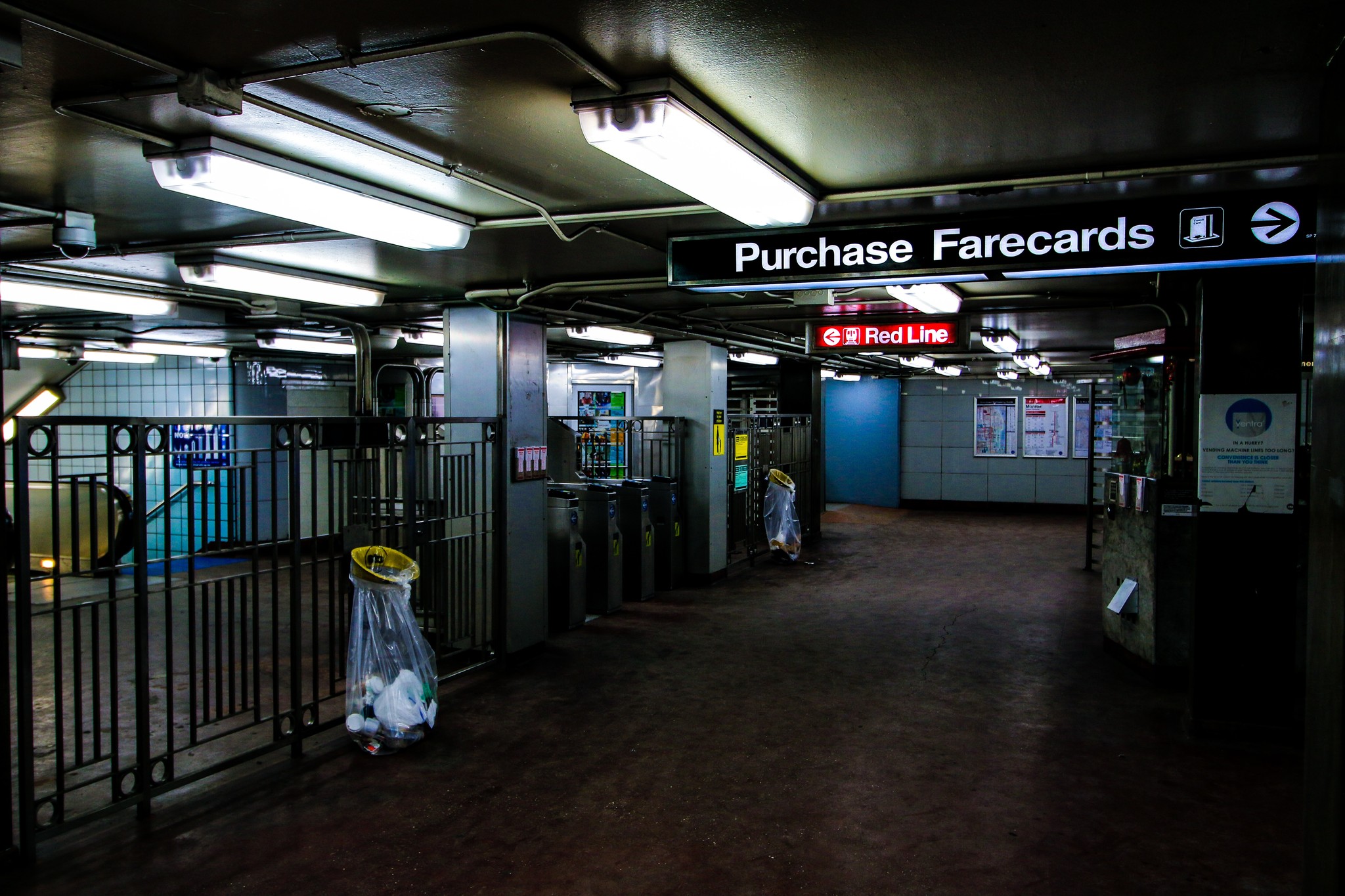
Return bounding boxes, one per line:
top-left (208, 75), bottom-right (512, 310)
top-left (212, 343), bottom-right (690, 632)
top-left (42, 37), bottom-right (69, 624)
top-left (16, 326), bottom-right (361, 366)
top-left (143, 142), bottom-right (476, 251)
top-left (567, 279), bottom-right (1055, 387)
top-left (569, 70), bottom-right (825, 234)
top-left (172, 246), bottom-right (391, 309)
